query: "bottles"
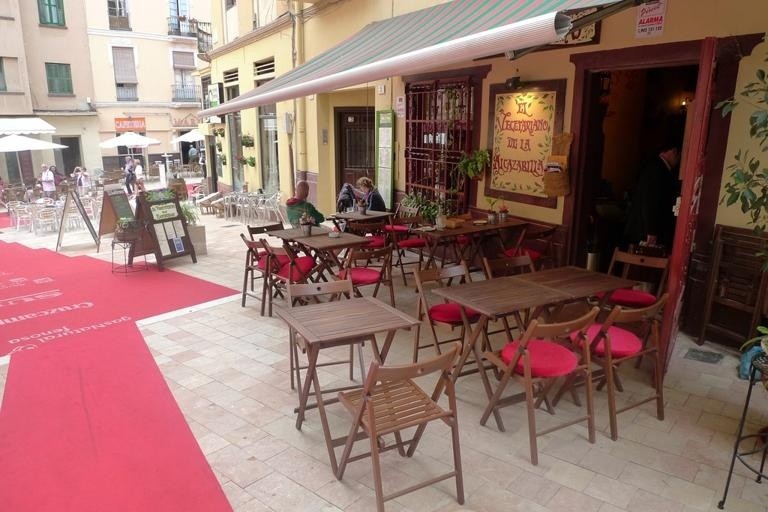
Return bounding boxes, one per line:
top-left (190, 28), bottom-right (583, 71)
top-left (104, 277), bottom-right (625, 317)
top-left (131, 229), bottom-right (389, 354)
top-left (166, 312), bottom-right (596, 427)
top-left (353, 198), bottom-right (357, 211)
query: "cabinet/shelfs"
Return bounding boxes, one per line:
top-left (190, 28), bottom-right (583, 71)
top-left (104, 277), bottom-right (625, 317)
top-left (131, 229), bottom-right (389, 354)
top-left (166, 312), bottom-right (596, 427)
top-left (111, 240), bottom-right (148, 274)
top-left (717, 353), bottom-right (768, 509)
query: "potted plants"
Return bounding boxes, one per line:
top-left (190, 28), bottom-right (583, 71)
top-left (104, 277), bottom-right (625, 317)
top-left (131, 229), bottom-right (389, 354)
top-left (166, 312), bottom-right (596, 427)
top-left (113, 214), bottom-right (144, 241)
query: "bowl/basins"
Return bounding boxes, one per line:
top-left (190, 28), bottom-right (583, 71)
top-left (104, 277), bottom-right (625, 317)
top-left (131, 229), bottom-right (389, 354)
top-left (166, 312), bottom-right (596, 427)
top-left (347, 207), bottom-right (353, 212)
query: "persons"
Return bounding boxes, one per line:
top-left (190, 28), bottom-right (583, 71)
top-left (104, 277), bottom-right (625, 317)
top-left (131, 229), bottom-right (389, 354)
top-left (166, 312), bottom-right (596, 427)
top-left (641, 145), bottom-right (681, 251)
top-left (348, 177), bottom-right (385, 212)
top-left (134, 159), bottom-right (145, 199)
top-left (124, 156), bottom-right (136, 196)
top-left (286, 181), bottom-right (344, 267)
top-left (37, 164), bottom-right (90, 203)
top-left (199, 149), bottom-right (207, 179)
top-left (189, 144), bottom-right (198, 162)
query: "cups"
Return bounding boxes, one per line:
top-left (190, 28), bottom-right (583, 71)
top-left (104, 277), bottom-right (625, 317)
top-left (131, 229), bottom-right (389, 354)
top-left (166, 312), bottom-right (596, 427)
top-left (301, 224), bottom-right (312, 237)
top-left (358, 205), bottom-right (366, 215)
top-left (646, 234), bottom-right (658, 245)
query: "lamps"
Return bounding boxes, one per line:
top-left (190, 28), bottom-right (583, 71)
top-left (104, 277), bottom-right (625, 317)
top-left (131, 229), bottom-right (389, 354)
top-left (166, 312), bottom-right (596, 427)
top-left (504, 77), bottom-right (522, 89)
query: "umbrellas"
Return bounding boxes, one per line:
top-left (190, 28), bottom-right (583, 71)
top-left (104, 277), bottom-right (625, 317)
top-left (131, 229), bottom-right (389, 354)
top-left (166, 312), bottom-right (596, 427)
top-left (99, 131), bottom-right (162, 159)
top-left (170, 128), bottom-right (205, 148)
top-left (0, 135), bottom-right (69, 186)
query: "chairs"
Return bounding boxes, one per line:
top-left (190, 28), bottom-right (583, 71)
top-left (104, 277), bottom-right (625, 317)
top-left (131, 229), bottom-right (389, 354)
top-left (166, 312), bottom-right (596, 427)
top-left (481, 255), bottom-right (551, 350)
top-left (607, 247), bottom-right (671, 369)
top-left (5, 192), bottom-right (103, 236)
top-left (285, 272), bottom-right (366, 419)
top-left (552, 294), bottom-right (670, 441)
top-left (474, 307), bottom-right (601, 465)
top-left (411, 259), bottom-right (501, 381)
top-left (222, 189), bottom-right (289, 225)
top-left (336, 340), bottom-right (464, 512)
top-left (698, 224), bottom-right (768, 350)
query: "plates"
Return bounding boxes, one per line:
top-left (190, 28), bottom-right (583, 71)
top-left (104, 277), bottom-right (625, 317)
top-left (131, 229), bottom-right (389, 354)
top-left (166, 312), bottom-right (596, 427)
top-left (472, 219), bottom-right (487, 225)
